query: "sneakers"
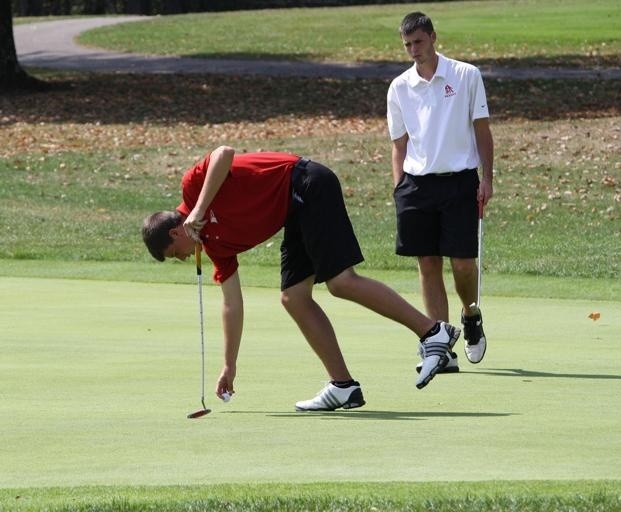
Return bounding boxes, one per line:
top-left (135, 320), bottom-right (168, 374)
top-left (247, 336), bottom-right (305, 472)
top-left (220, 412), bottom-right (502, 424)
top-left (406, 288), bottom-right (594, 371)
top-left (461, 307), bottom-right (486, 363)
top-left (295, 381), bottom-right (366, 411)
top-left (415, 320), bottom-right (461, 389)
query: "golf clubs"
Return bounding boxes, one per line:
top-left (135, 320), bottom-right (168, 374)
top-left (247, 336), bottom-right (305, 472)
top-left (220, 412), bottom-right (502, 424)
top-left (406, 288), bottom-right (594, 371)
top-left (469, 195), bottom-right (483, 321)
top-left (187, 231), bottom-right (211, 418)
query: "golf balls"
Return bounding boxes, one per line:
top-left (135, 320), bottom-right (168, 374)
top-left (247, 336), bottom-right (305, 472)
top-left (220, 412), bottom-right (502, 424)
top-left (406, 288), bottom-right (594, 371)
top-left (221, 393), bottom-right (230, 402)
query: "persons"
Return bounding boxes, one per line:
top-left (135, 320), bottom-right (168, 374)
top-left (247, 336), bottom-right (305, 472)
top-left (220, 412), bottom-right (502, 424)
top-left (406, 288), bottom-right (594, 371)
top-left (141, 146), bottom-right (461, 411)
top-left (386, 11), bottom-right (493, 374)
